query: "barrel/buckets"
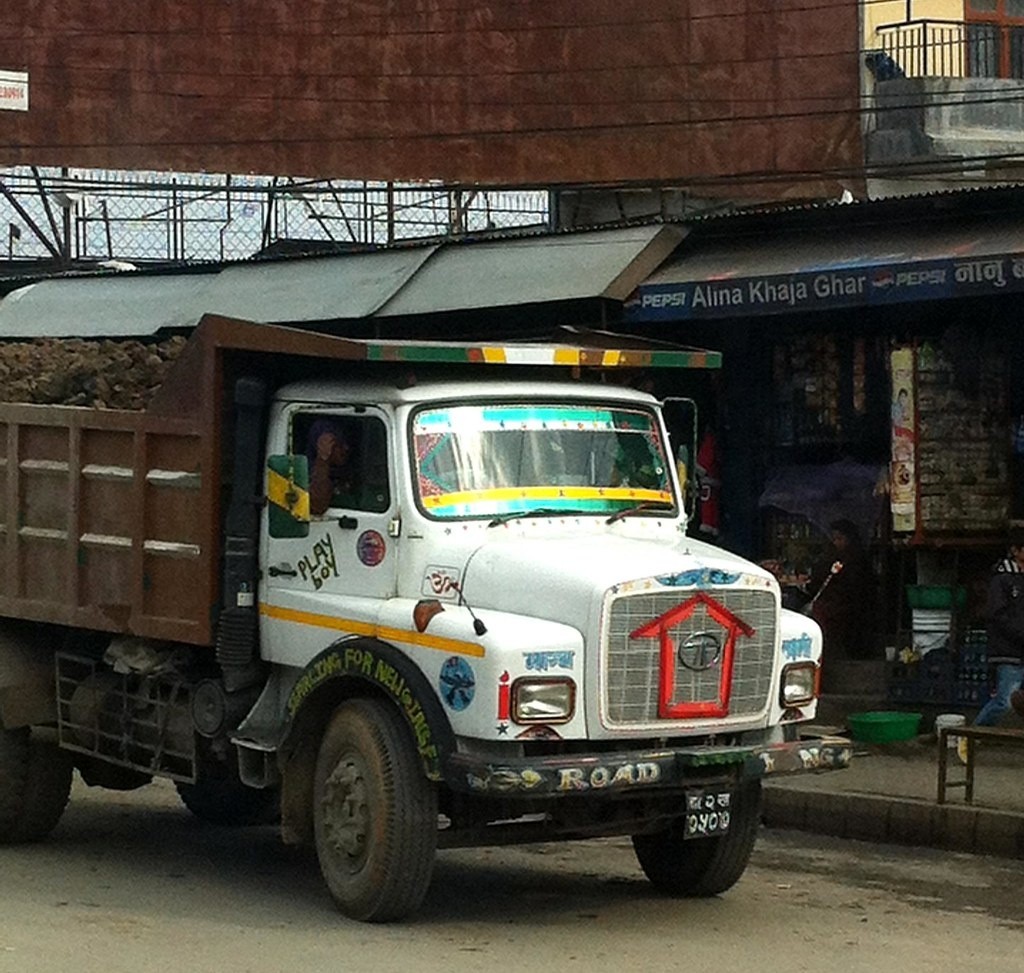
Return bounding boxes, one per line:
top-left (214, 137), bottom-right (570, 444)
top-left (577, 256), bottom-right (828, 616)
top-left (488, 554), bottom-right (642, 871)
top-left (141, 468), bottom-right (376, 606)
top-left (937, 714), bottom-right (966, 748)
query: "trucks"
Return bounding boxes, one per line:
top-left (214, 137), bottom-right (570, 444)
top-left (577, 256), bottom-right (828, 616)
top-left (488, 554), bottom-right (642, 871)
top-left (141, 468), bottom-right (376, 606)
top-left (0, 310), bottom-right (859, 924)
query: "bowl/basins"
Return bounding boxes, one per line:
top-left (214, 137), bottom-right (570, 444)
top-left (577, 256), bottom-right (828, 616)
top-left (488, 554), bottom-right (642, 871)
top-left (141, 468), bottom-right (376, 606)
top-left (848, 711), bottom-right (923, 744)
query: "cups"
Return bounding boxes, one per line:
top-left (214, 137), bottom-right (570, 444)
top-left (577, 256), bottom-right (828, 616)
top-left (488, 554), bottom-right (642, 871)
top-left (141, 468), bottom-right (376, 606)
top-left (886, 647), bottom-right (895, 662)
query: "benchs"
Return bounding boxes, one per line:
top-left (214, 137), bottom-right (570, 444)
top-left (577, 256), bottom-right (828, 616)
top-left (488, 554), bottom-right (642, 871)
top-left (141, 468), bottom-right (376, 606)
top-left (937, 725), bottom-right (1024, 805)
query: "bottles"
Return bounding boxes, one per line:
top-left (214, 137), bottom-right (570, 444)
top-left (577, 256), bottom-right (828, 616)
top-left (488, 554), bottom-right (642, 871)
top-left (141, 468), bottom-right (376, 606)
top-left (957, 629), bottom-right (988, 699)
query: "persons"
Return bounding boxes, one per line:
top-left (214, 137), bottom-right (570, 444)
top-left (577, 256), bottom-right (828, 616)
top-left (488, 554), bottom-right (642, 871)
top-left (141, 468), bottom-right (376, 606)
top-left (957, 526), bottom-right (1024, 763)
top-left (305, 420), bottom-right (377, 519)
top-left (814, 518), bottom-right (879, 660)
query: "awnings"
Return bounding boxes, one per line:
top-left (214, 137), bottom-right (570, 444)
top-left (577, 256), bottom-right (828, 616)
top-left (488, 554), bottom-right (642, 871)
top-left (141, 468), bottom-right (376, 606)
top-left (620, 209), bottom-right (1024, 323)
